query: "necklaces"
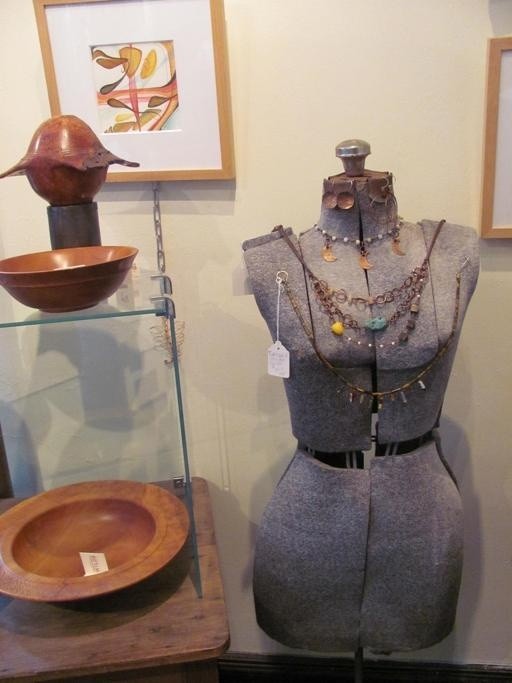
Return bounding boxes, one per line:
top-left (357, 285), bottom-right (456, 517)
top-left (268, 217), bottom-right (471, 409)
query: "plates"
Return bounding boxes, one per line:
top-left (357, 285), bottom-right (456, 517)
top-left (2, 480), bottom-right (189, 604)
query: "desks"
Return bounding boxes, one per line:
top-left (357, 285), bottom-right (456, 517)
top-left (1, 477), bottom-right (230, 681)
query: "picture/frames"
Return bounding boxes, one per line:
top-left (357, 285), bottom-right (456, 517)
top-left (481, 36), bottom-right (512, 240)
top-left (31, 1), bottom-right (235, 183)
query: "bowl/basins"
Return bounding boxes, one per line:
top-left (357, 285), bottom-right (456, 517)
top-left (1, 246), bottom-right (140, 313)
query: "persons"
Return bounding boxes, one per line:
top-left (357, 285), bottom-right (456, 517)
top-left (240, 171), bottom-right (483, 661)
